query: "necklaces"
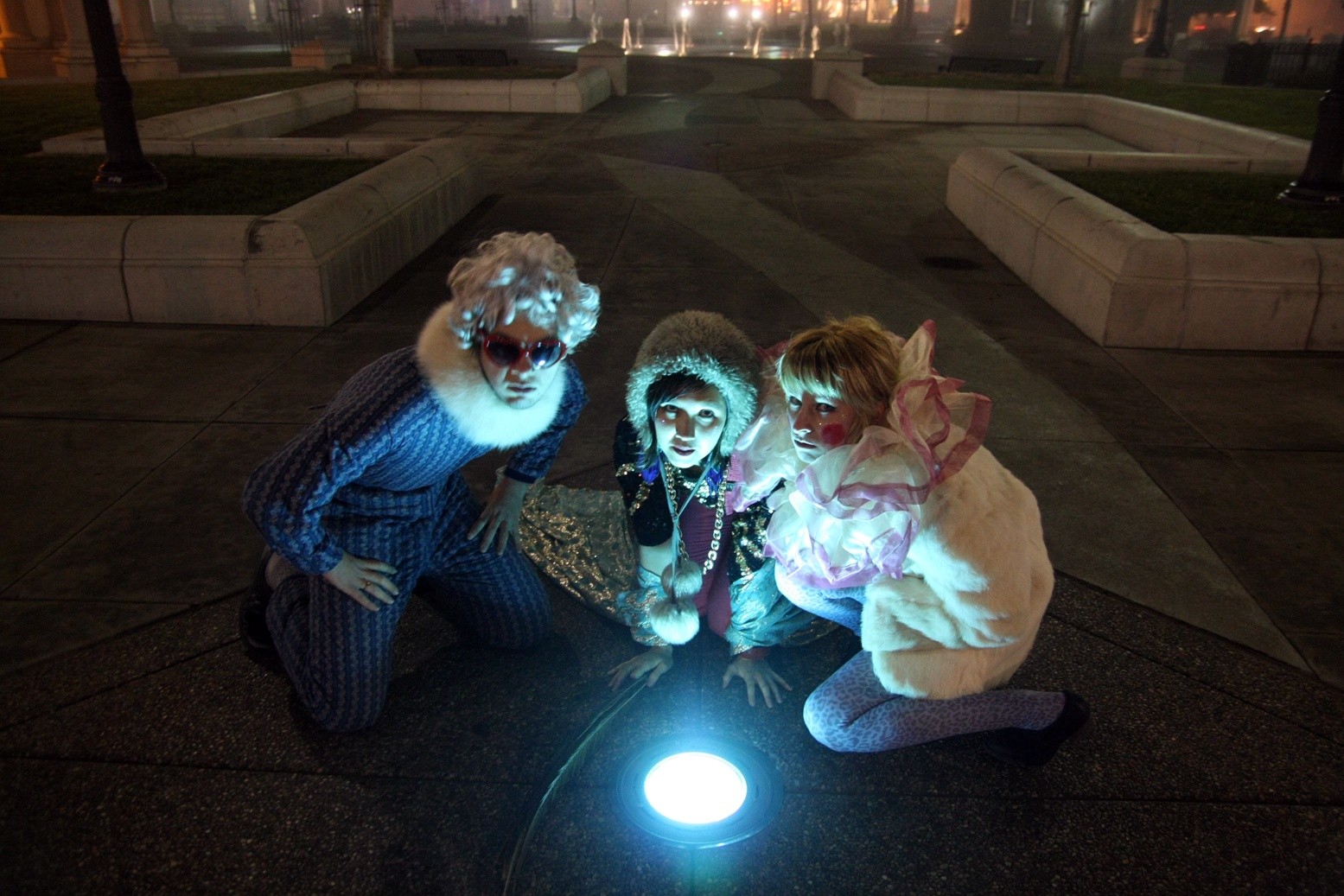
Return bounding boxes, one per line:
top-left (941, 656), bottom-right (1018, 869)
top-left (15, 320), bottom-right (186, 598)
top-left (664, 455), bottom-right (729, 575)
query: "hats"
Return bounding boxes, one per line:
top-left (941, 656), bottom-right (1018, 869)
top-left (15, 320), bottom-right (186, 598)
top-left (627, 312), bottom-right (762, 645)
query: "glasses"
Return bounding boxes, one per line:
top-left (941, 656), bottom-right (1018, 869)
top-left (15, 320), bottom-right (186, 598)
top-left (476, 327), bottom-right (568, 370)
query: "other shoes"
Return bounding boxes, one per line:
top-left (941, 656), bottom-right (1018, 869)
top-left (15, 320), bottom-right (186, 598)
top-left (983, 687), bottom-right (1091, 769)
top-left (237, 541), bottom-right (283, 652)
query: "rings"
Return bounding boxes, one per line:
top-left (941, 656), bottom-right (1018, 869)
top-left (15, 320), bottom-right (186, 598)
top-left (363, 580), bottom-right (370, 589)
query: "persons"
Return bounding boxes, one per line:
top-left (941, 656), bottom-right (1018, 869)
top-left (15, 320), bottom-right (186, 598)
top-left (731, 316), bottom-right (1097, 763)
top-left (519, 307), bottom-right (842, 709)
top-left (243, 235), bottom-right (602, 736)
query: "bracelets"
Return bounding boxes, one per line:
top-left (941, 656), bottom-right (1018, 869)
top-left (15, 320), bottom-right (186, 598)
top-left (738, 645), bottom-right (766, 659)
